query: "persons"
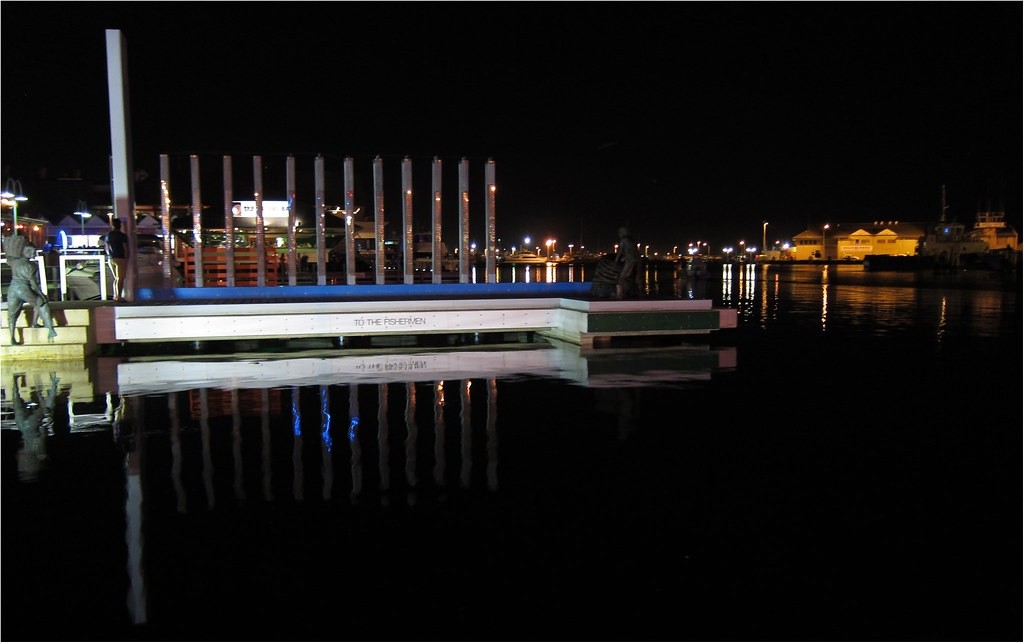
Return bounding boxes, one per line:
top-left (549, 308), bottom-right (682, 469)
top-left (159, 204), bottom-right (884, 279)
top-left (6, 243), bottom-right (56, 345)
top-left (103, 218), bottom-right (130, 302)
top-left (297, 250), bottom-right (310, 273)
top-left (614, 227), bottom-right (645, 301)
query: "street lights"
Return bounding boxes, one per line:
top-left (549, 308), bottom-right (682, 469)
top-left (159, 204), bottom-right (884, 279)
top-left (740, 241), bottom-right (745, 255)
top-left (823, 224), bottom-right (829, 258)
top-left (553, 240), bottom-right (556, 251)
top-left (673, 246), bottom-right (677, 254)
top-left (546, 240), bottom-right (552, 258)
top-left (703, 243), bottom-right (711, 256)
top-left (1, 177), bottom-right (29, 237)
top-left (697, 240), bottom-right (701, 253)
top-left (763, 222), bottom-right (768, 252)
top-left (644, 245), bottom-right (649, 256)
top-left (74, 200), bottom-right (92, 248)
